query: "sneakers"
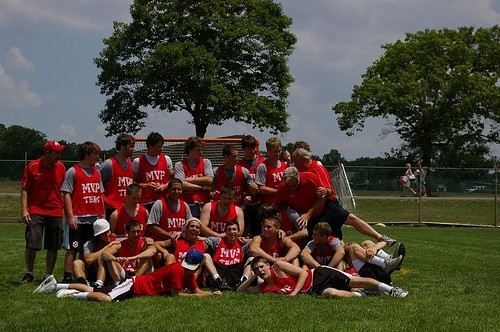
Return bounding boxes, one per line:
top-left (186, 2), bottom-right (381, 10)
top-left (55, 289), bottom-right (79, 299)
top-left (32, 275), bottom-right (57, 295)
top-left (352, 288), bottom-right (378, 297)
top-left (389, 288), bottom-right (409, 298)
top-left (378, 235), bottom-right (397, 247)
top-left (393, 243), bottom-right (406, 270)
top-left (384, 256), bottom-right (402, 274)
top-left (20, 273), bottom-right (34, 282)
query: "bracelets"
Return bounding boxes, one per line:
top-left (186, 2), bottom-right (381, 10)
top-left (200, 185), bottom-right (203, 191)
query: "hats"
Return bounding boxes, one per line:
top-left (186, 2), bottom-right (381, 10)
top-left (44, 141), bottom-right (64, 151)
top-left (182, 217), bottom-right (201, 237)
top-left (93, 219), bottom-right (110, 236)
top-left (181, 250), bottom-right (204, 270)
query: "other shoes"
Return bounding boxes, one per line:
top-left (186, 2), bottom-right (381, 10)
top-left (77, 277), bottom-right (89, 286)
top-left (94, 280), bottom-right (104, 288)
top-left (215, 277), bottom-right (229, 290)
top-left (236, 276), bottom-right (247, 291)
top-left (62, 273), bottom-right (74, 283)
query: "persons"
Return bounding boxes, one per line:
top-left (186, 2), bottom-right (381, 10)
top-left (171, 220), bottom-right (286, 290)
top-left (18, 140), bottom-right (67, 285)
top-left (146, 178), bottom-right (193, 268)
top-left (200, 183), bottom-right (244, 238)
top-left (243, 218), bottom-right (300, 285)
top-left (73, 219), bottom-right (154, 289)
top-left (94, 133), bottom-right (336, 238)
top-left (235, 256), bottom-right (410, 298)
top-left (102, 220), bottom-right (156, 284)
top-left (60, 141), bottom-right (105, 283)
top-left (152, 217), bottom-right (248, 294)
top-left (32, 250), bottom-right (222, 303)
top-left (110, 183), bottom-right (153, 239)
top-left (300, 222), bottom-right (345, 272)
top-left (399, 161), bottom-right (427, 197)
top-left (272, 166), bottom-right (397, 247)
top-left (344, 240), bottom-right (406, 287)
